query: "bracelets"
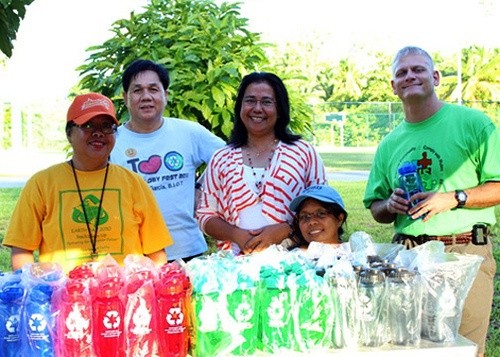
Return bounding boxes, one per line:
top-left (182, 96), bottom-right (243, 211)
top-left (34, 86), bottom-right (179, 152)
top-left (280, 219), bottom-right (296, 239)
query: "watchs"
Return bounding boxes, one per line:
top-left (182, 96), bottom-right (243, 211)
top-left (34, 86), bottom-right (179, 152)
top-left (454, 189), bottom-right (468, 209)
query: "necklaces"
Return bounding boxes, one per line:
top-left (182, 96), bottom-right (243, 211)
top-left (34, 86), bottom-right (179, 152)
top-left (246, 137), bottom-right (279, 194)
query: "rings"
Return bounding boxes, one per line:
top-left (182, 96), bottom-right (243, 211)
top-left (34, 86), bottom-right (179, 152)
top-left (426, 207), bottom-right (430, 211)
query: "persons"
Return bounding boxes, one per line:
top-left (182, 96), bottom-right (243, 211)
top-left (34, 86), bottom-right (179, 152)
top-left (287, 185), bottom-right (348, 250)
top-left (110, 59), bottom-right (228, 263)
top-left (362, 46), bottom-right (500, 357)
top-left (195, 71), bottom-right (328, 257)
top-left (2, 92), bottom-right (174, 270)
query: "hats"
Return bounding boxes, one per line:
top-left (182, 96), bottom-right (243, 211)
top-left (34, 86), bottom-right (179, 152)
top-left (67, 92), bottom-right (120, 126)
top-left (288, 184), bottom-right (350, 217)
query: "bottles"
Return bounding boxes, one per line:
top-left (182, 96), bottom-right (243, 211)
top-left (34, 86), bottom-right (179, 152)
top-left (313, 231), bottom-right (467, 349)
top-left (0, 267), bottom-right (53, 357)
top-left (257, 250), bottom-right (333, 354)
top-left (189, 257), bottom-right (257, 357)
top-left (58, 264), bottom-right (189, 357)
top-left (397, 164), bottom-right (425, 217)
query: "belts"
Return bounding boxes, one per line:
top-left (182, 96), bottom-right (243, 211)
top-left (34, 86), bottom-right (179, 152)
top-left (411, 231), bottom-right (474, 246)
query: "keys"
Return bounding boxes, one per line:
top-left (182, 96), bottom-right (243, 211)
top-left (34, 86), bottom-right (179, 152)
top-left (90, 252), bottom-right (98, 260)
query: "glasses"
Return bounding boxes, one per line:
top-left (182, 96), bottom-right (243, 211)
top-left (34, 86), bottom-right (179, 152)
top-left (69, 122), bottom-right (118, 134)
top-left (241, 95), bottom-right (277, 108)
top-left (295, 209), bottom-right (335, 222)
